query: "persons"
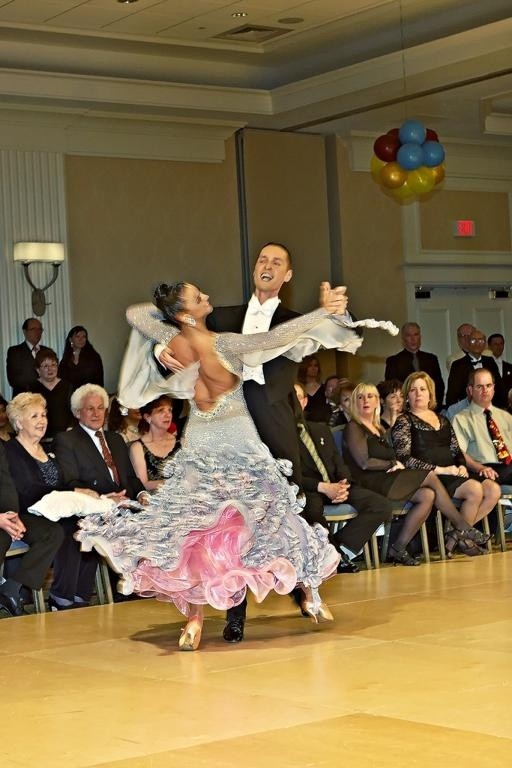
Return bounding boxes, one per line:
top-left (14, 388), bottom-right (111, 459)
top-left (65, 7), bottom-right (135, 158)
top-left (5, 391), bottom-right (99, 611)
top-left (57, 326), bottom-right (104, 398)
top-left (445, 331), bottom-right (504, 411)
top-left (376, 379), bottom-right (404, 430)
top-left (128, 396), bottom-right (185, 494)
top-left (292, 383), bottom-right (391, 574)
top-left (73, 280), bottom-right (341, 651)
top-left (52, 383), bottom-right (154, 513)
top-left (445, 323), bottom-right (502, 378)
top-left (487, 333), bottom-right (512, 412)
top-left (0, 393), bottom-right (18, 445)
top-left (22, 349), bottom-right (78, 451)
top-left (329, 378), bottom-right (357, 427)
top-left (1, 436), bottom-right (64, 615)
top-left (307, 376), bottom-right (340, 422)
top-left (149, 242), bottom-right (364, 643)
top-left (453, 368), bottom-right (512, 539)
top-left (384, 322), bottom-right (445, 407)
top-left (439, 369), bottom-right (472, 423)
top-left (298, 356), bottom-right (333, 422)
top-left (6, 318), bottom-right (58, 401)
top-left (391, 371), bottom-right (501, 560)
top-left (342, 383), bottom-right (494, 565)
top-left (107, 396), bottom-right (149, 443)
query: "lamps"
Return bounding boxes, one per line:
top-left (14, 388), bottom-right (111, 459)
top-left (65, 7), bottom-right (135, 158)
top-left (13, 242), bottom-right (65, 317)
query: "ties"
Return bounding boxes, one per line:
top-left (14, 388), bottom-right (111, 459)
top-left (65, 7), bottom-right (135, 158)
top-left (483, 408), bottom-right (512, 465)
top-left (297, 423), bottom-right (331, 484)
top-left (31, 345), bottom-right (37, 360)
top-left (412, 352), bottom-right (419, 372)
top-left (95, 431), bottom-right (120, 491)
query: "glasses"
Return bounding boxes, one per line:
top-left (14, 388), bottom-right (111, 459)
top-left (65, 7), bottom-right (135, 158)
top-left (24, 327), bottom-right (45, 332)
top-left (39, 362), bottom-right (58, 369)
top-left (468, 338), bottom-right (486, 344)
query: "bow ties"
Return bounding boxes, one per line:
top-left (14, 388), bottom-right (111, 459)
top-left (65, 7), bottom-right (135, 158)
top-left (249, 304), bottom-right (270, 317)
top-left (472, 359), bottom-right (482, 366)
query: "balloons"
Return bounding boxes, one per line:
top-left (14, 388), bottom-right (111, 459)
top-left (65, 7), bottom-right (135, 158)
top-left (408, 166), bottom-right (435, 194)
top-left (387, 127), bottom-right (402, 145)
top-left (393, 181), bottom-right (418, 206)
top-left (370, 153), bottom-right (389, 180)
top-left (398, 120), bottom-right (427, 145)
top-left (422, 141), bottom-right (445, 166)
top-left (381, 162), bottom-right (407, 189)
top-left (425, 126), bottom-right (438, 142)
top-left (373, 135), bottom-right (401, 162)
top-left (431, 165), bottom-right (446, 184)
top-left (397, 143), bottom-right (425, 170)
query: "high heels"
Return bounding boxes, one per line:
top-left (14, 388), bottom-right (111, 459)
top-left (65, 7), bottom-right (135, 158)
top-left (459, 540), bottom-right (489, 556)
top-left (455, 527), bottom-right (497, 550)
top-left (177, 615), bottom-right (202, 653)
top-left (443, 529), bottom-right (462, 558)
top-left (387, 544), bottom-right (420, 568)
top-left (301, 599), bottom-right (334, 623)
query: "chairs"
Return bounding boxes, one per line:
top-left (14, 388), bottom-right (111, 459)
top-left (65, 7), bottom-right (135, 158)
top-left (331, 424), bottom-right (431, 568)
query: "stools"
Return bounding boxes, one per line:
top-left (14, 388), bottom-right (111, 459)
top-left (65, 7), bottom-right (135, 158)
top-left (6, 539), bottom-right (45, 614)
top-left (322, 503), bottom-right (372, 570)
top-left (438, 498), bottom-right (492, 559)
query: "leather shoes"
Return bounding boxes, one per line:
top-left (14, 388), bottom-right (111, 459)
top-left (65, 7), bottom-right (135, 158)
top-left (1, 593), bottom-right (27, 620)
top-left (46, 593), bottom-right (76, 611)
top-left (77, 599), bottom-right (91, 608)
top-left (222, 616), bottom-right (242, 643)
top-left (335, 554), bottom-right (359, 574)
top-left (288, 586), bottom-right (309, 616)
